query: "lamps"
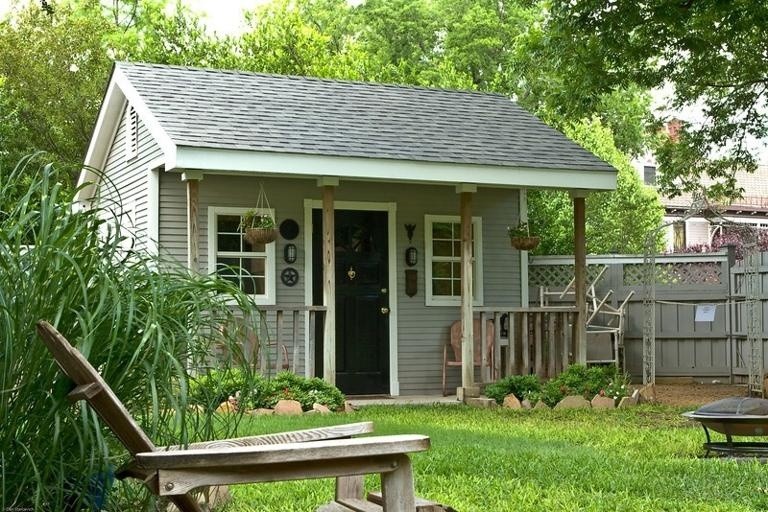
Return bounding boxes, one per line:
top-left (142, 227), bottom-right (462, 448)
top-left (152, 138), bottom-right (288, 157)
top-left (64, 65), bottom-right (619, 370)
top-left (406, 246), bottom-right (418, 266)
top-left (284, 243), bottom-right (297, 264)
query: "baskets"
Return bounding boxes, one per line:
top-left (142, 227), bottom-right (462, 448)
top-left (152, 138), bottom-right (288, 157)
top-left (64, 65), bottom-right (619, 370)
top-left (512, 237), bottom-right (540, 250)
top-left (241, 228), bottom-right (280, 246)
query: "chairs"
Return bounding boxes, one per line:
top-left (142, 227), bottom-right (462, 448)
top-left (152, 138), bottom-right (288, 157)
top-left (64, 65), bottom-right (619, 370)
top-left (36, 319), bottom-right (457, 512)
top-left (441, 317), bottom-right (495, 397)
